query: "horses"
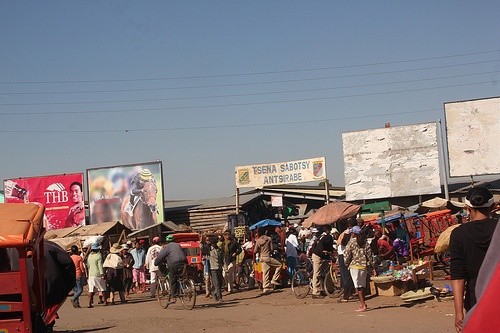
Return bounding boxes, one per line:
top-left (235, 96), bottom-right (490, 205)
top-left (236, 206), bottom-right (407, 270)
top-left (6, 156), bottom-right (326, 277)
top-left (121, 182), bottom-right (158, 230)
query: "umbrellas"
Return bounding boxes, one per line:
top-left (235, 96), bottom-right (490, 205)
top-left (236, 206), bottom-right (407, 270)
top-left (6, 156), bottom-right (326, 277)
top-left (303, 199), bottom-right (363, 233)
top-left (249, 219), bottom-right (282, 235)
top-left (405, 196), bottom-right (467, 215)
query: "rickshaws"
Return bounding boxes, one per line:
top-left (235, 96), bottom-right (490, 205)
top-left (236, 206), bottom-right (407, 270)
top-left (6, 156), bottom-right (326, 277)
top-left (0, 202), bottom-right (77, 333)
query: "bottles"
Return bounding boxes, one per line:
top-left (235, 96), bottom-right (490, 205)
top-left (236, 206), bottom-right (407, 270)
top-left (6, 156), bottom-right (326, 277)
top-left (419, 258), bottom-right (423, 265)
top-left (389, 260), bottom-right (394, 272)
top-left (424, 254), bottom-right (428, 262)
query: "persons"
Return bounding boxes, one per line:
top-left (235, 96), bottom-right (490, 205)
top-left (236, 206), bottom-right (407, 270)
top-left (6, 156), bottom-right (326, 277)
top-left (64, 245), bottom-right (85, 309)
top-left (100, 194), bottom-right (486, 313)
top-left (448, 185), bottom-right (500, 331)
top-left (64, 181), bottom-right (86, 229)
top-left (85, 242), bottom-right (106, 308)
top-left (124, 170), bottom-right (159, 215)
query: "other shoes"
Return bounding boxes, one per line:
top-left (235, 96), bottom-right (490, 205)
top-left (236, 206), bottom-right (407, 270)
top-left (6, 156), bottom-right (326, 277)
top-left (71, 299), bottom-right (81, 308)
top-left (355, 303), bottom-right (367, 312)
top-left (312, 292), bottom-right (327, 298)
top-left (337, 298), bottom-right (348, 303)
top-left (270, 280), bottom-right (282, 285)
top-left (170, 298), bottom-right (176, 302)
top-left (263, 288), bottom-right (273, 292)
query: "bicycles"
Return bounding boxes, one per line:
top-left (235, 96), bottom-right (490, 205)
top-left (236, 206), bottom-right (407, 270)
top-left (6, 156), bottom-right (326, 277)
top-left (291, 251), bottom-right (348, 300)
top-left (154, 262), bottom-right (197, 310)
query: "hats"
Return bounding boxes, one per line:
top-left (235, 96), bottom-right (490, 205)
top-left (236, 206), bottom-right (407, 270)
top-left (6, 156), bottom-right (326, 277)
top-left (152, 236), bottom-right (161, 243)
top-left (166, 235), bottom-right (174, 241)
top-left (91, 243), bottom-right (102, 249)
top-left (122, 244), bottom-right (129, 250)
top-left (126, 240), bottom-right (132, 245)
top-left (110, 243), bottom-right (124, 253)
top-left (351, 226), bottom-right (361, 234)
top-left (330, 228), bottom-right (340, 234)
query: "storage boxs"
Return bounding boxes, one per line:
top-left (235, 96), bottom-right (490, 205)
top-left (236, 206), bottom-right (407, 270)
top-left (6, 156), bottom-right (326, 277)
top-left (375, 282), bottom-right (401, 295)
